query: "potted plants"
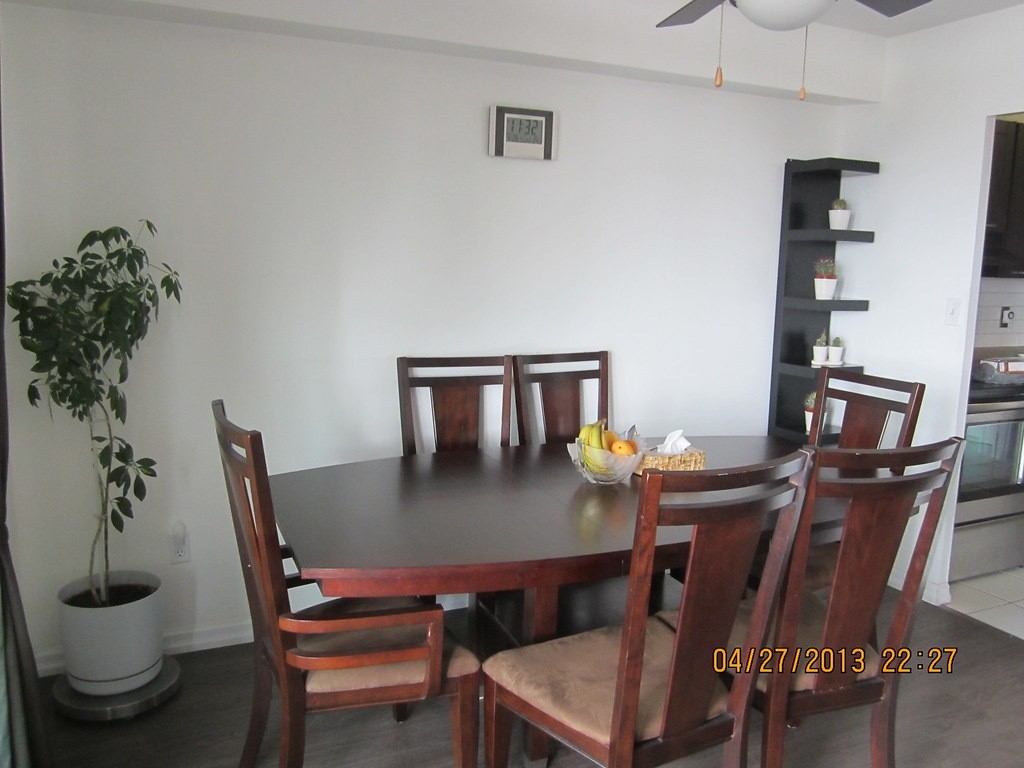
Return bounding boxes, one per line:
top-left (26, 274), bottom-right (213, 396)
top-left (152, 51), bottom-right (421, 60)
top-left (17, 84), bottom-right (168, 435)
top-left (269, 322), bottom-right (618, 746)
top-left (812, 328), bottom-right (844, 368)
top-left (828, 199), bottom-right (853, 230)
top-left (6, 216), bottom-right (181, 697)
top-left (804, 390), bottom-right (828, 431)
top-left (814, 259), bottom-right (838, 301)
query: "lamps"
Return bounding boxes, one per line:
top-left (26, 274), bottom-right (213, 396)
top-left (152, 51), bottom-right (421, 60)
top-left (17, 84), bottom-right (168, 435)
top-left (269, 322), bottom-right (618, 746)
top-left (735, 0), bottom-right (837, 32)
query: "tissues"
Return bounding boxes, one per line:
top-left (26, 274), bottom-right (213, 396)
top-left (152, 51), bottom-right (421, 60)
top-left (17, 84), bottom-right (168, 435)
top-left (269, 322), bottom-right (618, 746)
top-left (633, 430), bottom-right (706, 477)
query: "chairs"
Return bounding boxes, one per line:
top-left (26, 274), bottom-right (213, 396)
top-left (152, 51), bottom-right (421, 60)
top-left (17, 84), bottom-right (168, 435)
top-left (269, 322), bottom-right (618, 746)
top-left (211, 352), bottom-right (967, 768)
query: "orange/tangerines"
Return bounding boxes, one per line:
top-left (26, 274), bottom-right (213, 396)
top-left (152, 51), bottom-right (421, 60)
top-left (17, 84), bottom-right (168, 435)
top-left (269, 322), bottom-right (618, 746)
top-left (605, 430), bottom-right (637, 457)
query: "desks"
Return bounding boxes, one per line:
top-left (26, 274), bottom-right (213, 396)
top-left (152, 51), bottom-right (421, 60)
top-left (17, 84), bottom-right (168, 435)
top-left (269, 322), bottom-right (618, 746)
top-left (268, 436), bottom-right (920, 759)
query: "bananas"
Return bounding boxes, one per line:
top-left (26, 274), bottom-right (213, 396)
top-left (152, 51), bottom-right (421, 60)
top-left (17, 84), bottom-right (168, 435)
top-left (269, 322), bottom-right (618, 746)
top-left (578, 418), bottom-right (611, 473)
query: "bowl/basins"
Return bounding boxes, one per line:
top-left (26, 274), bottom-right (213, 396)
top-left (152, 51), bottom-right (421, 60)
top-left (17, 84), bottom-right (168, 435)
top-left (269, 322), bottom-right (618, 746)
top-left (567, 424), bottom-right (646, 485)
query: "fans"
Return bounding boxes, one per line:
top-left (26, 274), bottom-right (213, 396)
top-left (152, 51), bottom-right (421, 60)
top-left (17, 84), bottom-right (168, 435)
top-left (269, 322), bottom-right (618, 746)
top-left (656, 0), bottom-right (934, 28)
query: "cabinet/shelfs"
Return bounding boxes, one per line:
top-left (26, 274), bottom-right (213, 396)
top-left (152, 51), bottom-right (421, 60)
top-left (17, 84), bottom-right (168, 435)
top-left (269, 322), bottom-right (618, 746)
top-left (767, 157), bottom-right (880, 442)
